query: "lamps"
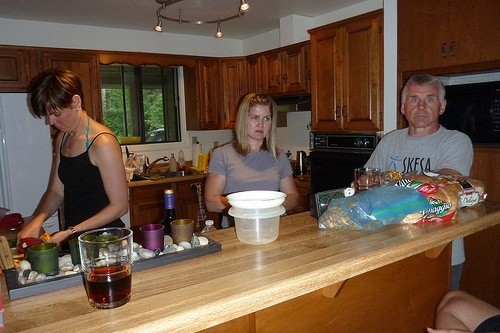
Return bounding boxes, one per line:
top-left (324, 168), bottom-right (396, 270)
top-left (154, 0), bottom-right (250, 39)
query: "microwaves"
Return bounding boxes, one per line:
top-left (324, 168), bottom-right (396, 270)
top-left (433, 70), bottom-right (500, 148)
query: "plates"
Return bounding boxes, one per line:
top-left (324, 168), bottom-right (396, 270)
top-left (227, 191), bottom-right (286, 209)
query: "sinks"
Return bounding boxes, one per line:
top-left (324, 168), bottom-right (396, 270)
top-left (155, 171), bottom-right (198, 178)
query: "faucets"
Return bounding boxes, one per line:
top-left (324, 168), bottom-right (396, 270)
top-left (144, 155), bottom-right (168, 176)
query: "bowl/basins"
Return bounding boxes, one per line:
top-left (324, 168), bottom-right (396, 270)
top-left (158, 166), bottom-right (169, 173)
top-left (228, 206), bottom-right (286, 245)
top-left (150, 167), bottom-right (158, 173)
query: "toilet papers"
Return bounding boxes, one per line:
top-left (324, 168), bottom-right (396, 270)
top-left (192, 144), bottom-right (202, 167)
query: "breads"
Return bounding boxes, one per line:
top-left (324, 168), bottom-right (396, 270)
top-left (395, 168), bottom-right (486, 223)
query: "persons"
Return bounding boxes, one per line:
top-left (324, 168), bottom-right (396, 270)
top-left (205, 93), bottom-right (300, 227)
top-left (427, 290), bottom-right (500, 333)
top-left (16, 68), bottom-right (129, 249)
top-left (362, 74), bottom-right (473, 291)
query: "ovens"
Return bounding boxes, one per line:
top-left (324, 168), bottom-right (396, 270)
top-left (310, 129), bottom-right (382, 196)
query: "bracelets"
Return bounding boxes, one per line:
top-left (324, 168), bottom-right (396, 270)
top-left (68, 226), bottom-right (76, 234)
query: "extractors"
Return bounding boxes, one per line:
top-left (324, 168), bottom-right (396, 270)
top-left (273, 96), bottom-right (311, 113)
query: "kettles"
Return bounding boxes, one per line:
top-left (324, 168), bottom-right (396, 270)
top-left (294, 150), bottom-right (307, 178)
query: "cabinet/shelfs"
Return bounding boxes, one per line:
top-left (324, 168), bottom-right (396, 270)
top-left (183, 9), bottom-right (385, 130)
top-left (0, 45), bottom-right (103, 138)
top-left (124, 177), bottom-right (226, 245)
top-left (397, 0), bottom-right (500, 71)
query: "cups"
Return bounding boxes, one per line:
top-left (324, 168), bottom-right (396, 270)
top-left (24, 243), bottom-right (58, 276)
top-left (78, 228), bottom-right (133, 310)
top-left (70, 239), bottom-right (80, 265)
top-left (170, 219), bottom-right (194, 244)
top-left (139, 224), bottom-right (164, 252)
top-left (354, 167), bottom-right (381, 192)
top-left (16, 237), bottom-right (42, 255)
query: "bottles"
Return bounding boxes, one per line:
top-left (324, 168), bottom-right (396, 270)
top-left (159, 188), bottom-right (180, 237)
top-left (169, 153), bottom-right (177, 173)
top-left (201, 220), bottom-right (215, 235)
top-left (177, 142), bottom-right (219, 171)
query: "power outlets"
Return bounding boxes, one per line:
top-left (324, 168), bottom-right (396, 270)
top-left (192, 136), bottom-right (197, 144)
